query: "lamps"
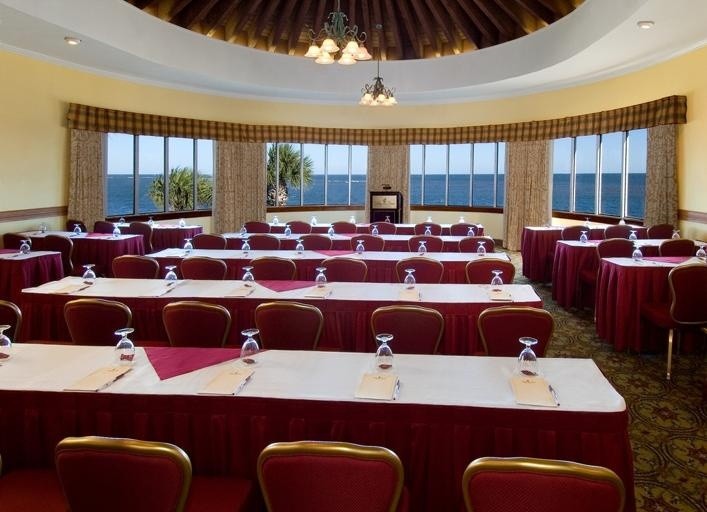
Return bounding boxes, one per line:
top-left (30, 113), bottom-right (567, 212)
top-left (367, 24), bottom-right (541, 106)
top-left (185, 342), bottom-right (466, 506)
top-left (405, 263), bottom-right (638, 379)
top-left (358, 23), bottom-right (399, 107)
top-left (302, 0), bottom-right (372, 65)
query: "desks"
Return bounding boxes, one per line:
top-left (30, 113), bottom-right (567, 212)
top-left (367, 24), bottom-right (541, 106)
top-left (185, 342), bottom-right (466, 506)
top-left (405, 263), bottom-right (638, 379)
top-left (0, 343), bottom-right (636, 509)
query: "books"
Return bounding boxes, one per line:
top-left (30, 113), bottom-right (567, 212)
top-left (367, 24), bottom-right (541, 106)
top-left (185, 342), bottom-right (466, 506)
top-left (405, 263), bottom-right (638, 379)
top-left (509, 375), bottom-right (556, 408)
top-left (63, 366), bottom-right (130, 391)
top-left (527, 217), bottom-right (707, 277)
top-left (2, 218), bottom-right (524, 305)
top-left (355, 369), bottom-right (397, 400)
top-left (199, 367), bottom-right (256, 397)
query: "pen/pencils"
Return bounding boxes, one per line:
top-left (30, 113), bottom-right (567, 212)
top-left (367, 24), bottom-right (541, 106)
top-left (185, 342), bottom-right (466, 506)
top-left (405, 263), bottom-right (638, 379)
top-left (233, 376), bottom-right (250, 395)
top-left (549, 385), bottom-right (560, 406)
top-left (95, 374), bottom-right (124, 392)
top-left (393, 380), bottom-right (399, 400)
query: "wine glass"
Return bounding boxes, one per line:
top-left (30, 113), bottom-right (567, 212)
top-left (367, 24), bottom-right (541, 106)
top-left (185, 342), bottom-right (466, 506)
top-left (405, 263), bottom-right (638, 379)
top-left (284, 225), bottom-right (292, 237)
top-left (240, 225), bottom-right (247, 237)
top-left (579, 216), bottom-right (707, 263)
top-left (241, 266), bottom-right (254, 287)
top-left (517, 335), bottom-right (541, 377)
top-left (112, 327), bottom-right (137, 365)
top-left (0, 322), bottom-right (14, 358)
top-left (242, 240), bottom-right (250, 256)
top-left (477, 241), bottom-right (486, 256)
top-left (328, 226), bottom-right (335, 237)
top-left (238, 328), bottom-right (261, 365)
top-left (112, 224), bottom-right (121, 238)
top-left (295, 240), bottom-right (304, 254)
top-left (403, 268), bottom-right (415, 289)
top-left (424, 227), bottom-right (431, 235)
top-left (117, 216), bottom-right (187, 227)
top-left (184, 239), bottom-right (194, 255)
top-left (38, 222), bottom-right (47, 234)
top-left (266, 216), bottom-right (465, 224)
top-left (418, 241), bottom-right (427, 255)
top-left (467, 227), bottom-right (474, 236)
top-left (164, 265), bottom-right (177, 287)
top-left (356, 240), bottom-right (365, 254)
top-left (314, 267), bottom-right (327, 288)
top-left (489, 269), bottom-right (503, 292)
top-left (74, 224), bottom-right (82, 235)
top-left (20, 240), bottom-right (30, 256)
top-left (374, 332), bottom-right (397, 371)
top-left (81, 263), bottom-right (96, 285)
top-left (372, 225), bottom-right (379, 236)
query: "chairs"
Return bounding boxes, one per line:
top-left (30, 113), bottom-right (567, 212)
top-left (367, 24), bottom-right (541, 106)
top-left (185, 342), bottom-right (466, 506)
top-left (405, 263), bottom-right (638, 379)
top-left (54, 434), bottom-right (254, 509)
top-left (2, 466), bottom-right (69, 510)
top-left (640, 262), bottom-right (706, 381)
top-left (1, 211), bottom-right (546, 356)
top-left (519, 222), bottom-right (706, 355)
top-left (476, 305), bottom-right (555, 358)
top-left (256, 439), bottom-right (412, 510)
top-left (461, 455), bottom-right (625, 510)
